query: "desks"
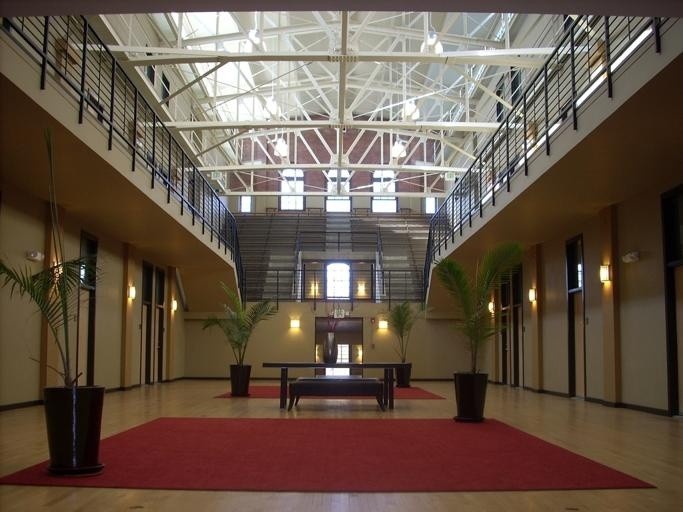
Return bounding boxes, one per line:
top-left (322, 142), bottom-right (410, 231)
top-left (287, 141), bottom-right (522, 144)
top-left (263, 363), bottom-right (410, 409)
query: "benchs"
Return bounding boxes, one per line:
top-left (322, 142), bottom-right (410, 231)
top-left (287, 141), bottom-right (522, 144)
top-left (287, 376), bottom-right (386, 412)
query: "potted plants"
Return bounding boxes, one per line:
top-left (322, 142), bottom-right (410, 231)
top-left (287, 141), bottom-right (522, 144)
top-left (0, 126), bottom-right (107, 479)
top-left (200, 279), bottom-right (281, 398)
top-left (432, 241), bottom-right (526, 423)
top-left (373, 300), bottom-right (431, 388)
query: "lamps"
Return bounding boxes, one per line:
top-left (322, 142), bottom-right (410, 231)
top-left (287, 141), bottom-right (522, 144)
top-left (249, 31), bottom-right (292, 162)
top-left (390, 34), bottom-right (443, 159)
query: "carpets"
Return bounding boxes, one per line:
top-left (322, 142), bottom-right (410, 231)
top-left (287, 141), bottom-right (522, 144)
top-left (1, 416), bottom-right (656, 492)
top-left (213, 385), bottom-right (447, 399)
